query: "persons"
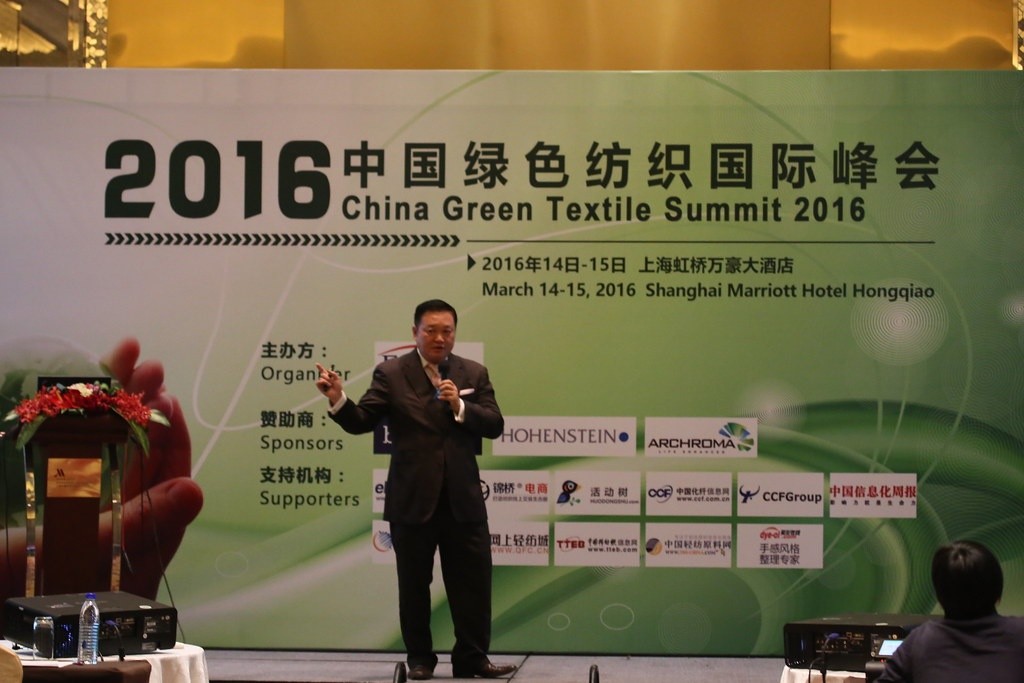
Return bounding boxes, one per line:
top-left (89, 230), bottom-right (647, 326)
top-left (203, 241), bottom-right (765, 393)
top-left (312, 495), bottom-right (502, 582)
top-left (0, 337), bottom-right (203, 596)
top-left (876, 540), bottom-right (1024, 682)
top-left (316, 299), bottom-right (516, 681)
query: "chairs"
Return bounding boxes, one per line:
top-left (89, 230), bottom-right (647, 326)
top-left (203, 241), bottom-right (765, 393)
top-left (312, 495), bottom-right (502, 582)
top-left (0, 644), bottom-right (24, 683)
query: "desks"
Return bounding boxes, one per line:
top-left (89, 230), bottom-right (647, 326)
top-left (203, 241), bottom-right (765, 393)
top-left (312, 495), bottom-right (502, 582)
top-left (0, 639), bottom-right (209, 683)
top-left (780, 665), bottom-right (866, 683)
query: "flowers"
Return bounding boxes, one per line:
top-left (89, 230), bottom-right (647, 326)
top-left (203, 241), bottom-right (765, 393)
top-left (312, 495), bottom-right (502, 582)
top-left (6, 379), bottom-right (171, 458)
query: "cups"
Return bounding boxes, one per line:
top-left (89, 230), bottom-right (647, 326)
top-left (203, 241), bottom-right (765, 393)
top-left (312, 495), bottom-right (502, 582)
top-left (33, 616), bottom-right (53, 660)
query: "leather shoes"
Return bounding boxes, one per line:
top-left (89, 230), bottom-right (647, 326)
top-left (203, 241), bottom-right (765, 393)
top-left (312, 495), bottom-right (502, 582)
top-left (451, 656), bottom-right (515, 679)
top-left (407, 661), bottom-right (433, 680)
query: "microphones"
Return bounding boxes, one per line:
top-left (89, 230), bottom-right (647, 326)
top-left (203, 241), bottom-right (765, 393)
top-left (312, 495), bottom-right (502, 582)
top-left (438, 360), bottom-right (451, 417)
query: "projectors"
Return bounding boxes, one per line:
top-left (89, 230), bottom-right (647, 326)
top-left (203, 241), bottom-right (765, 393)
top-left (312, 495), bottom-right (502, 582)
top-left (783, 613), bottom-right (929, 672)
top-left (2, 590), bottom-right (178, 659)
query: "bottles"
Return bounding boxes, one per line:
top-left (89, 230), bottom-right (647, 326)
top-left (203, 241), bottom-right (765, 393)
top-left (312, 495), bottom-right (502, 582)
top-left (77, 593), bottom-right (100, 665)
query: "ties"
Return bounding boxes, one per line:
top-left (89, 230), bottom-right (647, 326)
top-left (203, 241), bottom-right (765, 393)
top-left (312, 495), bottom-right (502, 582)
top-left (430, 363), bottom-right (441, 389)
